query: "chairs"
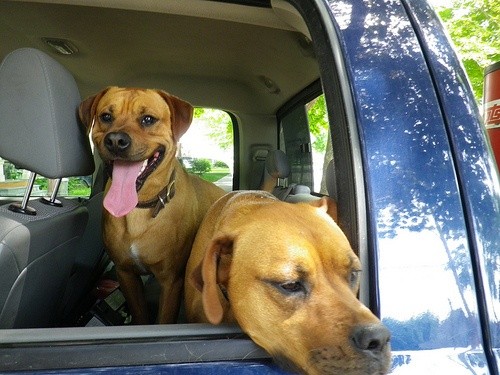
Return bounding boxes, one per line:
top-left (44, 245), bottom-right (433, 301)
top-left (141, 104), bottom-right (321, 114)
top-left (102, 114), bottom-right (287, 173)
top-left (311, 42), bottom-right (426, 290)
top-left (0, 47), bottom-right (115, 327)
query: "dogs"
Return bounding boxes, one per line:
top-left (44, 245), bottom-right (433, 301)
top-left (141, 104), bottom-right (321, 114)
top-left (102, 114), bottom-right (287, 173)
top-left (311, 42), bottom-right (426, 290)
top-left (182, 190), bottom-right (392, 375)
top-left (75, 86), bottom-right (228, 325)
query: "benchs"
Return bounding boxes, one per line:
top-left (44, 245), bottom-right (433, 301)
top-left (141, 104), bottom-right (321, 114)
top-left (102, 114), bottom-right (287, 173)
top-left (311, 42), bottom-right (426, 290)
top-left (263, 151), bottom-right (338, 214)
